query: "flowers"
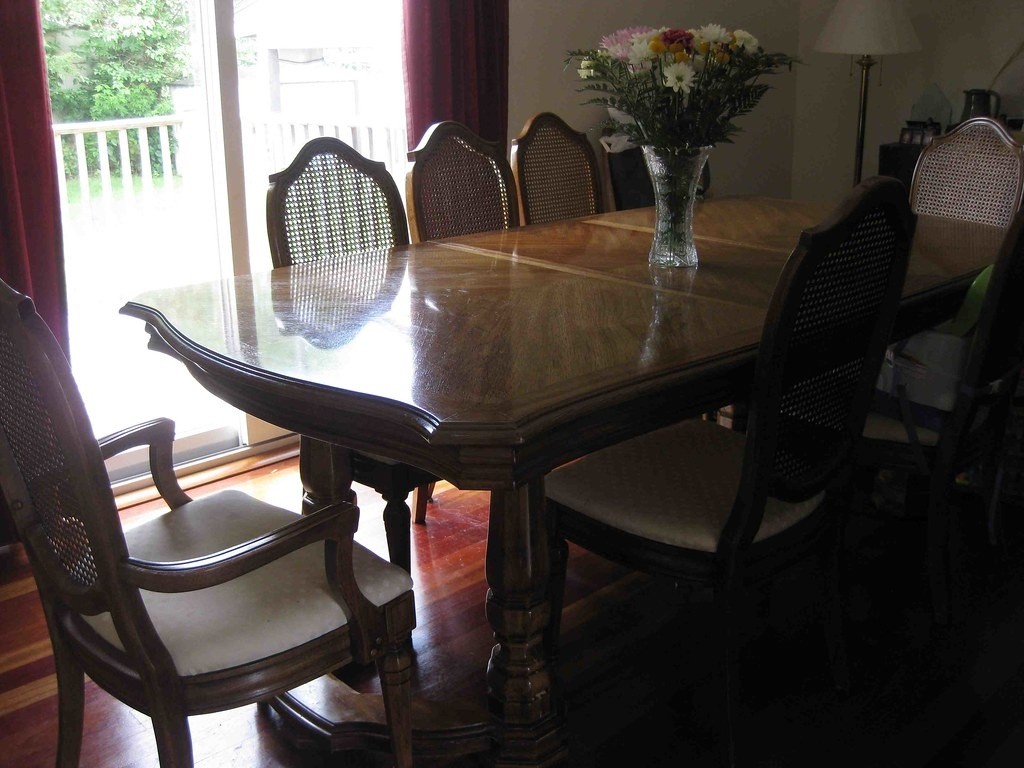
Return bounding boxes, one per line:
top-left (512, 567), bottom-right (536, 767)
top-left (563, 24), bottom-right (815, 265)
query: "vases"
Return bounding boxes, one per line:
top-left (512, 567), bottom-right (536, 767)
top-left (639, 144), bottom-right (713, 269)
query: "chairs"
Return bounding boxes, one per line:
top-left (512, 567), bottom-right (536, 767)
top-left (908, 113), bottom-right (1024, 229)
top-left (536, 174), bottom-right (918, 753)
top-left (407, 122), bottom-right (522, 501)
top-left (267, 138), bottom-right (449, 663)
top-left (515, 111), bottom-right (603, 229)
top-left (795, 208), bottom-right (1023, 627)
top-left (0, 277), bottom-right (419, 766)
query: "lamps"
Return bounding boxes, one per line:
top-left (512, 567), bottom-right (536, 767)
top-left (813, 0), bottom-right (923, 188)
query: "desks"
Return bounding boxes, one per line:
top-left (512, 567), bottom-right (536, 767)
top-left (126, 190), bottom-right (1017, 768)
